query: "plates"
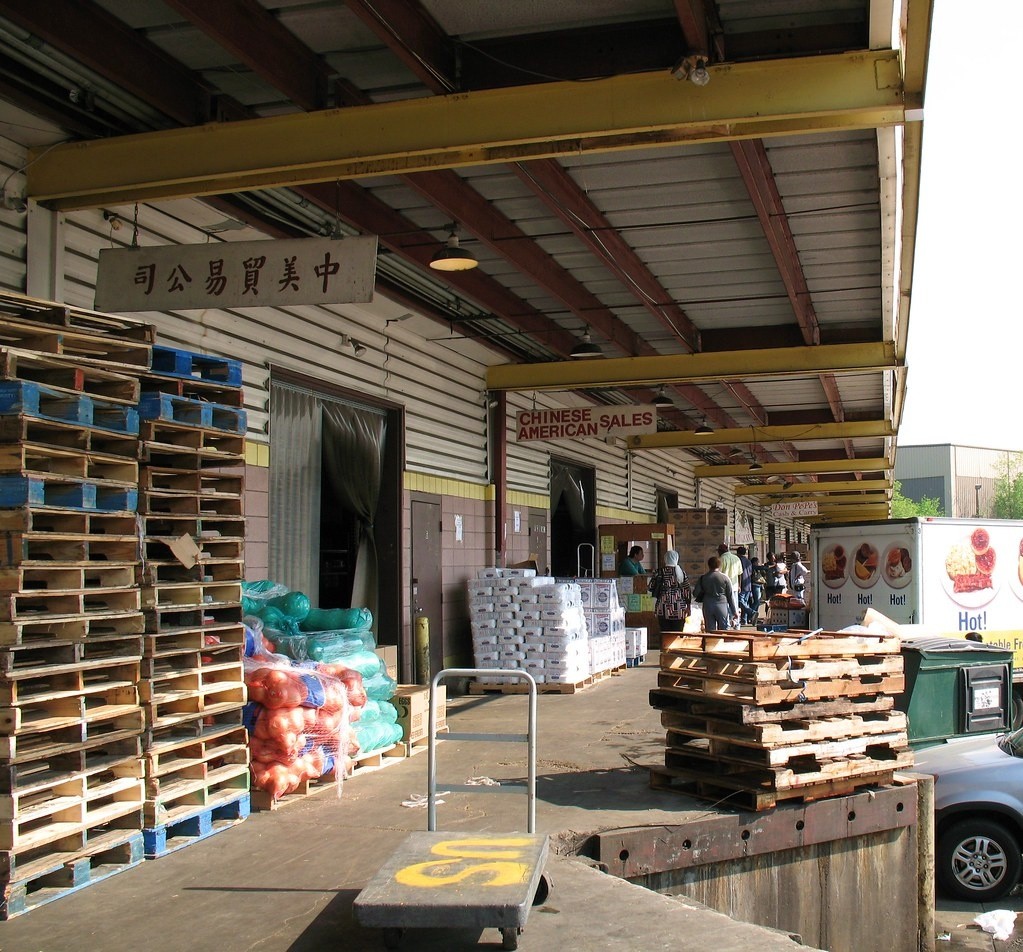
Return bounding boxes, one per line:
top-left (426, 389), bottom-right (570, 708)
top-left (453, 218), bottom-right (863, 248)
top-left (937, 552), bottom-right (1001, 608)
top-left (821, 543), bottom-right (848, 588)
top-left (880, 540), bottom-right (912, 588)
top-left (848, 543), bottom-right (880, 588)
top-left (1007, 550), bottom-right (1023, 602)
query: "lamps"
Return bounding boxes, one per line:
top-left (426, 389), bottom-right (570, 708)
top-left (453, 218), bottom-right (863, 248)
top-left (650, 385), bottom-right (675, 407)
top-left (679, 51), bottom-right (710, 85)
top-left (694, 416), bottom-right (715, 435)
top-left (729, 448), bottom-right (793, 490)
top-left (430, 221), bottom-right (478, 275)
top-left (343, 335), bottom-right (366, 360)
top-left (571, 323), bottom-right (605, 357)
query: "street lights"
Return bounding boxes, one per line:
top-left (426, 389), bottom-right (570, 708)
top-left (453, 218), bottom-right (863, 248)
top-left (975, 483), bottom-right (983, 518)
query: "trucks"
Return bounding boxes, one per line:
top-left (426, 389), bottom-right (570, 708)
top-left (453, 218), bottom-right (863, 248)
top-left (808, 516), bottom-right (1023, 731)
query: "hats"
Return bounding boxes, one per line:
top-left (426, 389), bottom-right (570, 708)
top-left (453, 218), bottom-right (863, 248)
top-left (716, 544), bottom-right (728, 552)
top-left (737, 547), bottom-right (746, 555)
top-left (751, 557), bottom-right (759, 564)
top-left (791, 551), bottom-right (800, 561)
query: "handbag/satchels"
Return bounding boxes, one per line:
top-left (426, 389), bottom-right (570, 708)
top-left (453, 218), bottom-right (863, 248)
top-left (693, 575), bottom-right (705, 603)
top-left (652, 568), bottom-right (664, 597)
top-left (751, 567), bottom-right (766, 584)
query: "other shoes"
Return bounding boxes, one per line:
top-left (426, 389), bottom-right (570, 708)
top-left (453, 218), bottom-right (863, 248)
top-left (741, 621), bottom-right (745, 624)
top-left (752, 611), bottom-right (759, 626)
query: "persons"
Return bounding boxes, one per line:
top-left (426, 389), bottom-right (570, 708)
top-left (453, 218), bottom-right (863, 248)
top-left (618, 546), bottom-right (647, 576)
top-left (692, 544), bottom-right (810, 633)
top-left (964, 632), bottom-right (983, 643)
top-left (649, 550), bottom-right (692, 632)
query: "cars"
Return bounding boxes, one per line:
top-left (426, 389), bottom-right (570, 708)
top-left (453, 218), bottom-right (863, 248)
top-left (894, 723), bottom-right (1022, 903)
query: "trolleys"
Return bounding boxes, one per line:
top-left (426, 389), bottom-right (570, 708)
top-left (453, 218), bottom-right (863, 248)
top-left (352, 667), bottom-right (549, 952)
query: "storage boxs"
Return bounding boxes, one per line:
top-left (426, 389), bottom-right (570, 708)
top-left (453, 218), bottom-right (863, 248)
top-left (372, 646), bottom-right (446, 743)
top-left (665, 508), bottom-right (729, 585)
